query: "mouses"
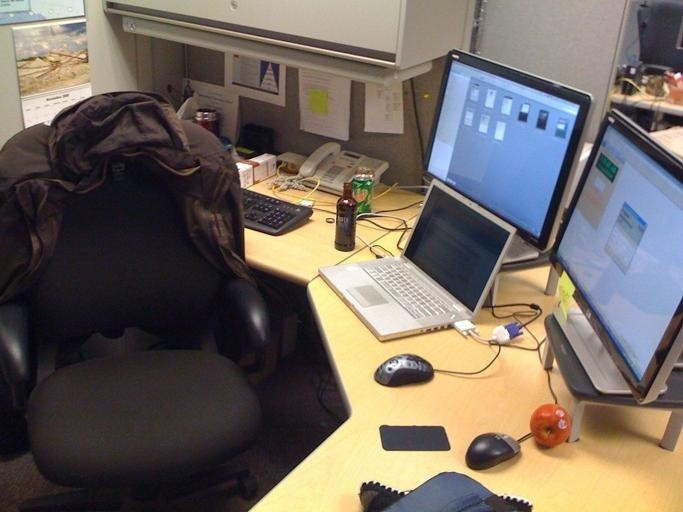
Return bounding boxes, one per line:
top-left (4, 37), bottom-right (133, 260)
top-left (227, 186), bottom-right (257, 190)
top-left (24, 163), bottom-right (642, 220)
top-left (373, 350), bottom-right (436, 388)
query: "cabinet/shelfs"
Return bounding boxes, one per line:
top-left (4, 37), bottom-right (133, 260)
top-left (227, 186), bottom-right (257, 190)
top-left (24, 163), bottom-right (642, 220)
top-left (99, 0), bottom-right (479, 86)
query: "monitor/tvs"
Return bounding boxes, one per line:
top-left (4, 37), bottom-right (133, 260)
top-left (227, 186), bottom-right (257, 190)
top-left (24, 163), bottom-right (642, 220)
top-left (546, 107), bottom-right (683, 407)
top-left (422, 47), bottom-right (595, 256)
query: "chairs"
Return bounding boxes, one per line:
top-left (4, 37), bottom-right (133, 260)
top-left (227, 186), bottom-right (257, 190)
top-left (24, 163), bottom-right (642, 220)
top-left (1, 117), bottom-right (273, 512)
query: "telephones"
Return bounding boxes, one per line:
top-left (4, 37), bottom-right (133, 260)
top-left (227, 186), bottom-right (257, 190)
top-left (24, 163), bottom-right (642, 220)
top-left (297, 140), bottom-right (391, 197)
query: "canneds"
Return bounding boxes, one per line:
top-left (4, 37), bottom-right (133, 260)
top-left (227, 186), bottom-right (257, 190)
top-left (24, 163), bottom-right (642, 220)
top-left (351, 175), bottom-right (375, 215)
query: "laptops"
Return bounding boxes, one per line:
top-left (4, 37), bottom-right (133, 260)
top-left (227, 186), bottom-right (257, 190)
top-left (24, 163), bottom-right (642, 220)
top-left (316, 177), bottom-right (519, 342)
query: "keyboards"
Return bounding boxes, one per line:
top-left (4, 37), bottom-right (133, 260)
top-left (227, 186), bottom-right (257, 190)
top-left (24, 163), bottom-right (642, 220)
top-left (239, 185), bottom-right (312, 233)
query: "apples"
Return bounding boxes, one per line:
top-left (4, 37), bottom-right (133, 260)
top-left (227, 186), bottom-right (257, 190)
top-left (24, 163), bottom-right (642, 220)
top-left (530, 405), bottom-right (572, 447)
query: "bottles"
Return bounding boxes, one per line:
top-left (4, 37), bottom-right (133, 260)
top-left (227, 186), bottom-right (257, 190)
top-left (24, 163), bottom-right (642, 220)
top-left (195, 107), bottom-right (220, 139)
top-left (335, 183), bottom-right (356, 252)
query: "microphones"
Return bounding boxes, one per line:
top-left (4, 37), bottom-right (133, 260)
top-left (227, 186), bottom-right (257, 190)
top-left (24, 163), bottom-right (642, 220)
top-left (466, 431), bottom-right (522, 471)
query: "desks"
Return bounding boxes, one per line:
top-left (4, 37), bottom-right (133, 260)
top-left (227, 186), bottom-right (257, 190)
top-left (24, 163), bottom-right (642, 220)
top-left (236, 165), bottom-right (681, 511)
